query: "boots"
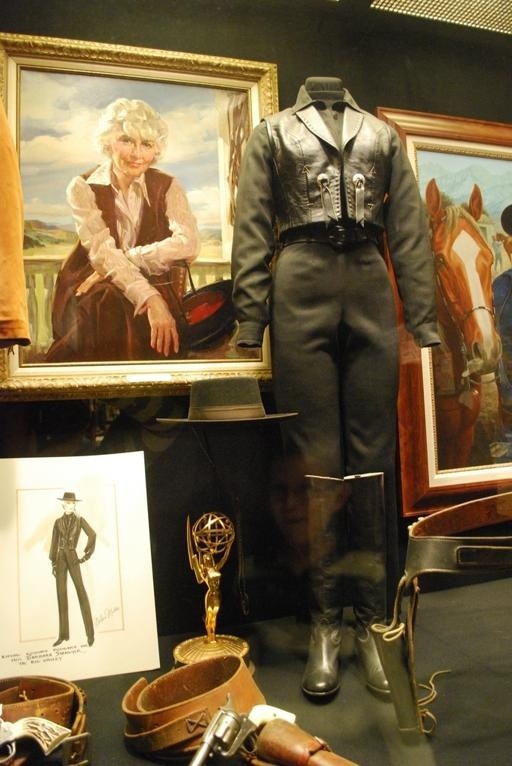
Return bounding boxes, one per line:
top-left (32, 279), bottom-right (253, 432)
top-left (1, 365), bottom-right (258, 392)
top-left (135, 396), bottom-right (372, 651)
top-left (300, 475), bottom-right (347, 700)
top-left (344, 472), bottom-right (392, 701)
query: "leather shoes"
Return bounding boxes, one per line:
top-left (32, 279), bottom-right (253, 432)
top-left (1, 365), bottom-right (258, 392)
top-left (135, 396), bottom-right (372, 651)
top-left (53, 637), bottom-right (69, 646)
top-left (87, 635), bottom-right (95, 645)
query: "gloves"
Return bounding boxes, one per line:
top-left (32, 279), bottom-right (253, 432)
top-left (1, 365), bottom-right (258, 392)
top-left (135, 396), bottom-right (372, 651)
top-left (78, 555), bottom-right (88, 564)
top-left (52, 561), bottom-right (57, 577)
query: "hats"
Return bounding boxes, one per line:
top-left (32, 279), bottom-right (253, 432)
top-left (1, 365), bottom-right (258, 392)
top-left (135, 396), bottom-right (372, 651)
top-left (57, 491), bottom-right (82, 503)
top-left (154, 376), bottom-right (299, 428)
top-left (182, 280), bottom-right (238, 352)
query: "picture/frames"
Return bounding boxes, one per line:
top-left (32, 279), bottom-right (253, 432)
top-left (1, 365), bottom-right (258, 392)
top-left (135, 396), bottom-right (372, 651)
top-left (377, 100), bottom-right (510, 522)
top-left (0, 25), bottom-right (284, 391)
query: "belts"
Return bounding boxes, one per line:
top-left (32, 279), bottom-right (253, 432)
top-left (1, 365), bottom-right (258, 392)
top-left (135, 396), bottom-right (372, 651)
top-left (120, 653), bottom-right (267, 762)
top-left (0, 674), bottom-right (90, 765)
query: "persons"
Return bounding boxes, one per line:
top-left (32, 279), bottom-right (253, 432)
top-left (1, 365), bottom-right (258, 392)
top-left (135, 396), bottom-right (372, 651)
top-left (234, 74), bottom-right (440, 700)
top-left (49, 98), bottom-right (200, 358)
top-left (493, 204), bottom-right (512, 435)
top-left (492, 236), bottom-right (505, 273)
top-left (49, 493), bottom-right (97, 647)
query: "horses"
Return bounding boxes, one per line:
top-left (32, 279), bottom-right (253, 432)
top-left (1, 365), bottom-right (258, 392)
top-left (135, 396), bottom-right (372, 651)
top-left (424, 176), bottom-right (503, 471)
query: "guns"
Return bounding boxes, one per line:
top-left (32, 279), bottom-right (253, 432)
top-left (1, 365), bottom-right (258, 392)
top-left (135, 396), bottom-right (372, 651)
top-left (189, 692), bottom-right (258, 766)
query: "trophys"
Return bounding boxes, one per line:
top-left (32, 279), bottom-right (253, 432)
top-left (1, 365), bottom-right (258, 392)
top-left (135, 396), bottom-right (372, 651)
top-left (172, 510), bottom-right (255, 684)
